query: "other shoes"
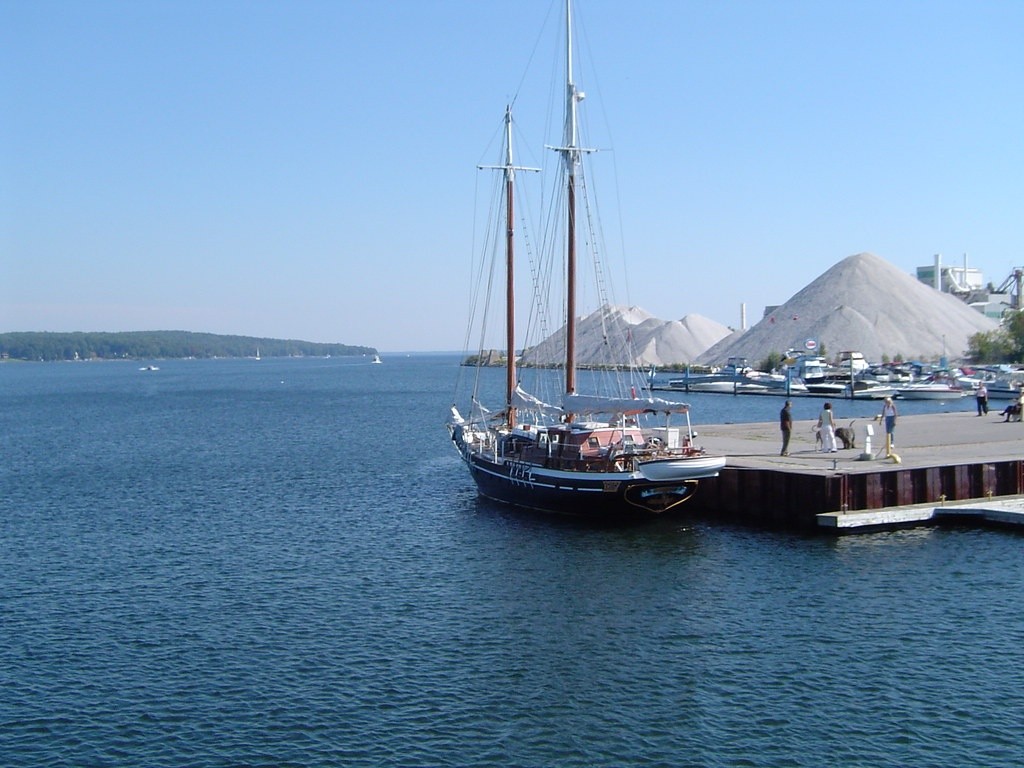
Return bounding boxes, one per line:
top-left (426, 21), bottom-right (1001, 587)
top-left (1000, 413), bottom-right (1004, 416)
top-left (831, 451), bottom-right (838, 453)
top-left (891, 443), bottom-right (894, 448)
top-left (1004, 419), bottom-right (1009, 422)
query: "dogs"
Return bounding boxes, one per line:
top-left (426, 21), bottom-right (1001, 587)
top-left (834, 420), bottom-right (856, 449)
top-left (811, 423), bottom-right (836, 452)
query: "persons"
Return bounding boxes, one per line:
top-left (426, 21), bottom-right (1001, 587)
top-left (976, 382), bottom-right (1024, 423)
top-left (780, 400), bottom-right (793, 456)
top-left (821, 403), bottom-right (837, 453)
top-left (879, 397), bottom-right (897, 448)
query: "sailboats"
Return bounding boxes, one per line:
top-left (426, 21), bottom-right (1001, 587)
top-left (444, 1), bottom-right (728, 523)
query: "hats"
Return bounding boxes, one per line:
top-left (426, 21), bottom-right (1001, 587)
top-left (1019, 387), bottom-right (1024, 393)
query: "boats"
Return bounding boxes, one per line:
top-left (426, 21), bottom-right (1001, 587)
top-left (645, 349), bottom-right (1024, 400)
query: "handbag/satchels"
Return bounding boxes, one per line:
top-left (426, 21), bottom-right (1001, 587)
top-left (984, 405), bottom-right (988, 412)
